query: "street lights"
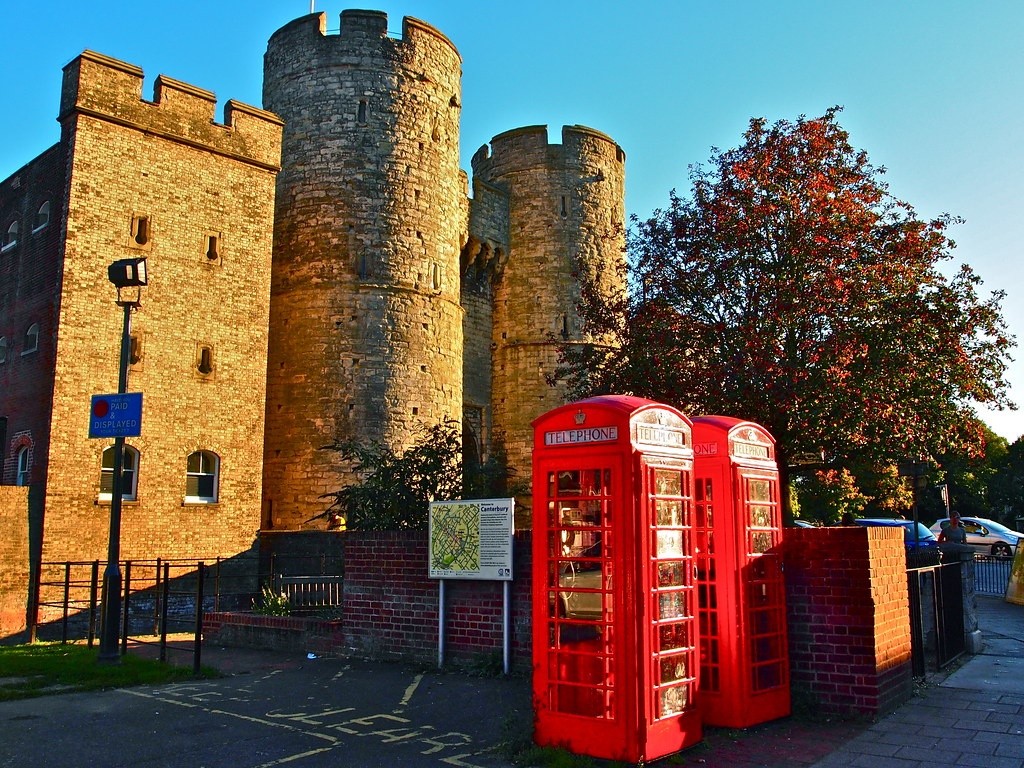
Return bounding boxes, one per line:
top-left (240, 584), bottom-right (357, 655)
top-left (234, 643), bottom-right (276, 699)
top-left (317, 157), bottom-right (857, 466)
top-left (97, 257), bottom-right (151, 662)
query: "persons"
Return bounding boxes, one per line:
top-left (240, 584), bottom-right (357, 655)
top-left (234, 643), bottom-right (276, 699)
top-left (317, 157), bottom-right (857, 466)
top-left (938, 511), bottom-right (968, 546)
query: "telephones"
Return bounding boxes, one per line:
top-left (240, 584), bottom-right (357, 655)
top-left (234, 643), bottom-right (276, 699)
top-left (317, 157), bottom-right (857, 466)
top-left (548, 507), bottom-right (584, 561)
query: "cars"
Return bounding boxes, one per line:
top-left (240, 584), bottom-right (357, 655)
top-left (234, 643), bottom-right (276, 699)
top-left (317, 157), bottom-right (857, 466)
top-left (929, 516), bottom-right (1024, 561)
top-left (828, 518), bottom-right (940, 567)
top-left (793, 519), bottom-right (818, 528)
